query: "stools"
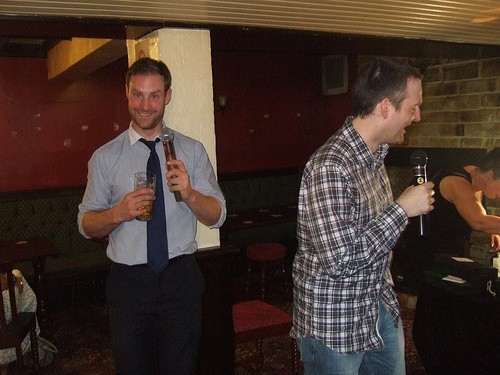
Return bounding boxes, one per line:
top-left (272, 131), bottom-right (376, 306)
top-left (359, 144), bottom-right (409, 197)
top-left (232, 299), bottom-right (300, 375)
top-left (244, 242), bottom-right (294, 303)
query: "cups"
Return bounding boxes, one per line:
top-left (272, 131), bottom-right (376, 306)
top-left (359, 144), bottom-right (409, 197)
top-left (134, 172), bottom-right (156, 222)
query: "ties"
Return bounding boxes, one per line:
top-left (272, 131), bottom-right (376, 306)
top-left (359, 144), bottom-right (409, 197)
top-left (138, 138), bottom-right (169, 274)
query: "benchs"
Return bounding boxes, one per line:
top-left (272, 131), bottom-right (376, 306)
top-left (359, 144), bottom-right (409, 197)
top-left (218, 166), bottom-right (412, 257)
top-left (0, 185), bottom-right (108, 304)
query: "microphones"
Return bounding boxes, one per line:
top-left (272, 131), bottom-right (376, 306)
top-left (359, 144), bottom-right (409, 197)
top-left (409, 151), bottom-right (429, 238)
top-left (160, 127), bottom-right (183, 202)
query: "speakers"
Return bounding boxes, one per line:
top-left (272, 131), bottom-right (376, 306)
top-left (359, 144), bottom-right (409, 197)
top-left (317, 54), bottom-right (348, 96)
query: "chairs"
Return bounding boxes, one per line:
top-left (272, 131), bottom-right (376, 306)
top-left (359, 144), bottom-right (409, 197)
top-left (0, 259), bottom-right (41, 375)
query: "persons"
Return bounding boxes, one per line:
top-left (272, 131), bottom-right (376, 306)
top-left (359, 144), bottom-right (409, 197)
top-left (290, 56), bottom-right (435, 375)
top-left (409, 147), bottom-right (500, 347)
top-left (77, 57), bottom-right (227, 375)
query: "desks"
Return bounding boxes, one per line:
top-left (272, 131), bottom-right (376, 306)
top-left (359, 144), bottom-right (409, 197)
top-left (0, 235), bottom-right (62, 343)
top-left (412, 269), bottom-right (500, 375)
